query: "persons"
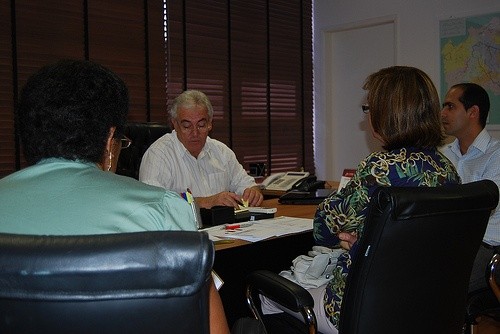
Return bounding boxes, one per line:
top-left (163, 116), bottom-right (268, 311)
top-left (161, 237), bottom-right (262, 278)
top-left (0, 58), bottom-right (233, 334)
top-left (264, 67), bottom-right (500, 334)
top-left (138, 90), bottom-right (264, 211)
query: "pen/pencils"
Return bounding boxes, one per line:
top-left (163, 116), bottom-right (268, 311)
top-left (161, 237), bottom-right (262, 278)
top-left (233, 199), bottom-right (245, 207)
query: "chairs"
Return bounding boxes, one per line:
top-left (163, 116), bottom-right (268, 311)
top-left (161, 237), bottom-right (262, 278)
top-left (246, 179), bottom-right (500, 334)
top-left (115, 123), bottom-right (172, 182)
top-left (0, 230), bottom-right (215, 334)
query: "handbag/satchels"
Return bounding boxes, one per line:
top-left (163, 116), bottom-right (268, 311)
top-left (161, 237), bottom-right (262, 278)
top-left (290, 243), bottom-right (347, 288)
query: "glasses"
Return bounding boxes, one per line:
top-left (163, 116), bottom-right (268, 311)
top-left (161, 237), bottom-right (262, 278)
top-left (361, 103), bottom-right (371, 114)
top-left (176, 118), bottom-right (213, 135)
top-left (112, 133), bottom-right (132, 149)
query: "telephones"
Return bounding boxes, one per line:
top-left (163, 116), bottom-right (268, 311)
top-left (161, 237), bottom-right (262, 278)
top-left (278, 175), bottom-right (336, 205)
top-left (259, 172), bottom-right (309, 191)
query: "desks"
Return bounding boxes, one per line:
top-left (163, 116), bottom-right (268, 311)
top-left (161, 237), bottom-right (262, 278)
top-left (213, 181), bottom-right (340, 252)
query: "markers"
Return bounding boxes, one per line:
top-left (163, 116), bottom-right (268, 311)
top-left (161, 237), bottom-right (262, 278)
top-left (226, 224), bottom-right (250, 229)
top-left (181, 188), bottom-right (204, 229)
